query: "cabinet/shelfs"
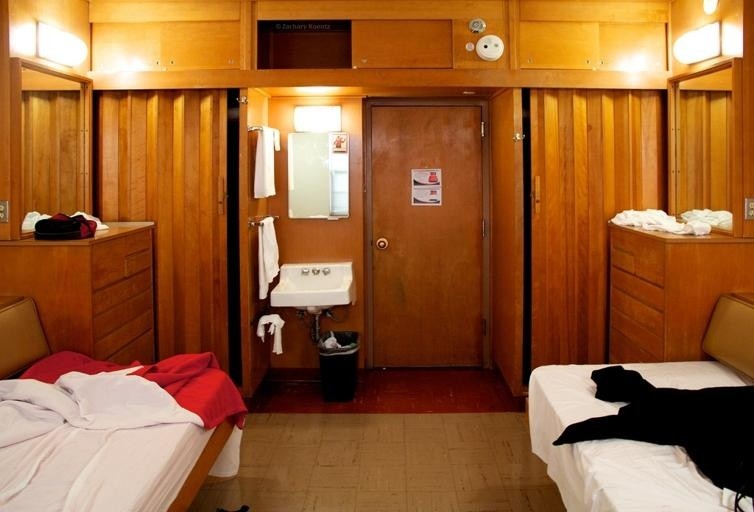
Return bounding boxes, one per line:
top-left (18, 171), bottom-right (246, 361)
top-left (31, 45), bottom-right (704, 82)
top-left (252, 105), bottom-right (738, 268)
top-left (13, 220), bottom-right (159, 371)
top-left (92, 88), bottom-right (251, 405)
top-left (518, 86), bottom-right (667, 385)
top-left (252, 19), bottom-right (455, 74)
top-left (597, 219), bottom-right (744, 366)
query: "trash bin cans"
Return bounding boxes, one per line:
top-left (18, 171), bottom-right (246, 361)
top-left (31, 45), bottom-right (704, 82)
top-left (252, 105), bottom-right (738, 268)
top-left (316, 329), bottom-right (362, 404)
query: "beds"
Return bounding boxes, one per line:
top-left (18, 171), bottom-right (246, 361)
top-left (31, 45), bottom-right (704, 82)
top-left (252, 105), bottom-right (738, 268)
top-left (0, 294), bottom-right (243, 511)
top-left (526, 290), bottom-right (754, 512)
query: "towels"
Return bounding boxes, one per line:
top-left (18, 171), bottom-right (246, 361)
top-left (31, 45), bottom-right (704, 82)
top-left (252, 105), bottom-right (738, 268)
top-left (252, 126), bottom-right (277, 200)
top-left (609, 207), bottom-right (712, 237)
top-left (256, 216), bottom-right (281, 300)
top-left (255, 313), bottom-right (285, 356)
top-left (682, 204), bottom-right (733, 233)
top-left (272, 128), bottom-right (281, 152)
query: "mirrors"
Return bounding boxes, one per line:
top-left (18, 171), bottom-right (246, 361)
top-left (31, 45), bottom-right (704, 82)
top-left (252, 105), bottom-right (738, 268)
top-left (8, 54), bottom-right (92, 218)
top-left (665, 58), bottom-right (745, 220)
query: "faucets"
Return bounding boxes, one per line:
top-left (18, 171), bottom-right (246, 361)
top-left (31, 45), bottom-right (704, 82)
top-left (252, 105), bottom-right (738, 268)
top-left (311, 267), bottom-right (320, 276)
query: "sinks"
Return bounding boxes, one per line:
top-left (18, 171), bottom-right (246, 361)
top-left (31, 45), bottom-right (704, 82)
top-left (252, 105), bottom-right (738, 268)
top-left (270, 262), bottom-right (355, 307)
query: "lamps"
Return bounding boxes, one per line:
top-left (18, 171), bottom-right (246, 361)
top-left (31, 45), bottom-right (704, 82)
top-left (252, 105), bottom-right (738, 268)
top-left (34, 21), bottom-right (90, 70)
top-left (674, 21), bottom-right (723, 65)
top-left (290, 105), bottom-right (342, 133)
